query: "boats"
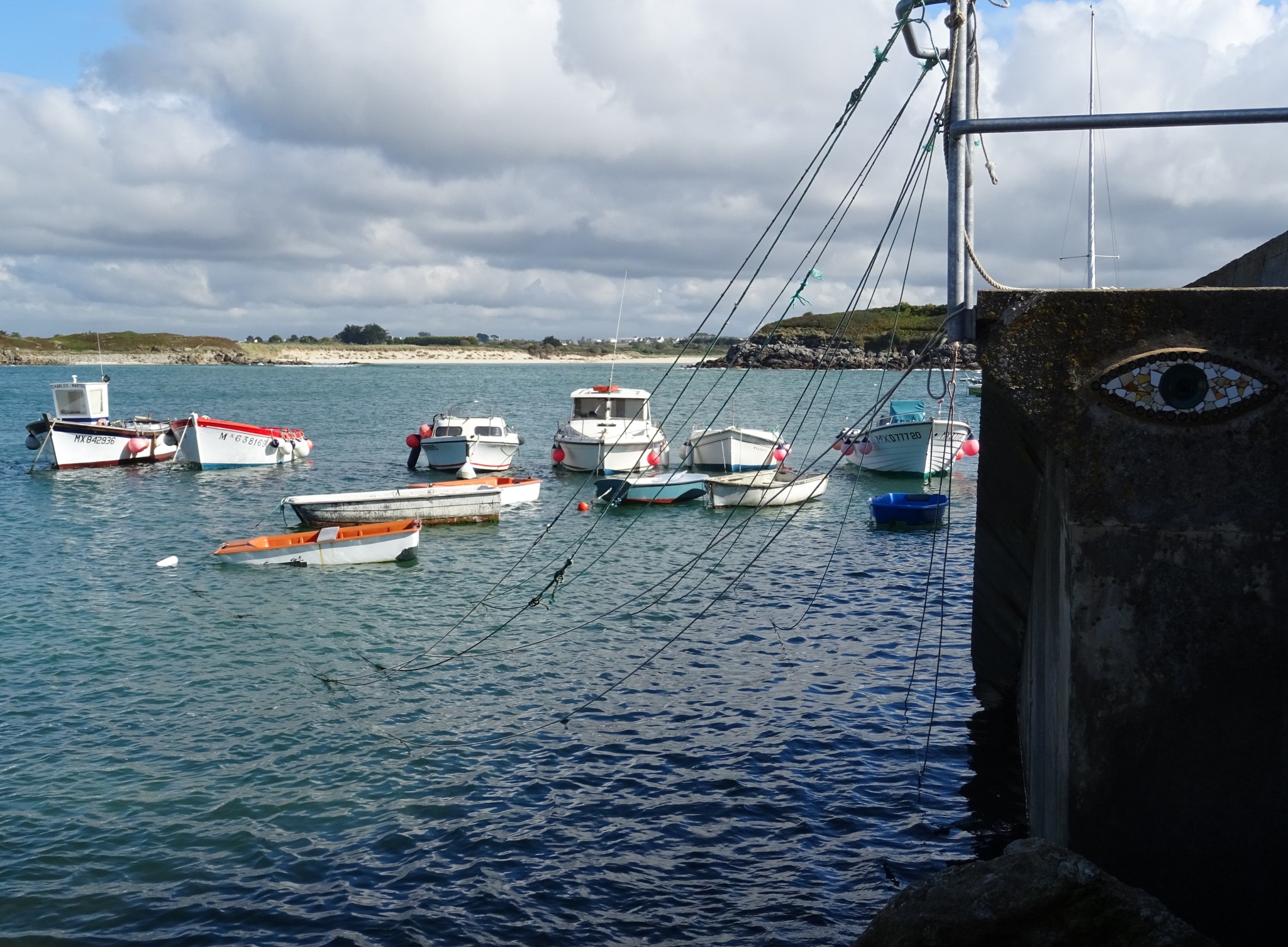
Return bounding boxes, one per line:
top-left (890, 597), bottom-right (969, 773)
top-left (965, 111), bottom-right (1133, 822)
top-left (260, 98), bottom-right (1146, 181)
top-left (593, 473), bottom-right (708, 505)
top-left (26, 332), bottom-right (182, 471)
top-left (406, 476), bottom-right (544, 506)
top-left (406, 400), bottom-right (525, 475)
top-left (703, 468), bottom-right (830, 509)
top-left (836, 400), bottom-right (972, 480)
top-left (170, 411), bottom-right (313, 472)
top-left (866, 494), bottom-right (949, 525)
top-left (283, 484), bottom-right (502, 526)
top-left (959, 374), bottom-right (983, 396)
top-left (551, 384), bottom-right (670, 475)
top-left (212, 517), bottom-right (423, 567)
top-left (677, 397), bottom-right (791, 471)
